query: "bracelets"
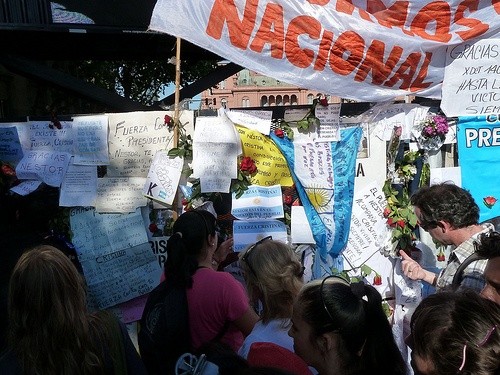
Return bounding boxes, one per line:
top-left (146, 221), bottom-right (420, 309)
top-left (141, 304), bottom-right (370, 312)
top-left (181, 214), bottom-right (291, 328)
top-left (212, 256), bottom-right (219, 267)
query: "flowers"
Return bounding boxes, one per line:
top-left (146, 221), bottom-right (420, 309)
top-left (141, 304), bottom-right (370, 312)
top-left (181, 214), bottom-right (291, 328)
top-left (418, 113), bottom-right (448, 189)
top-left (164, 109), bottom-right (257, 211)
top-left (382, 127), bottom-right (417, 254)
top-left (436, 252), bottom-right (445, 261)
top-left (338, 263), bottom-right (383, 295)
top-left (274, 98), bottom-right (329, 141)
top-left (483, 196), bottom-right (499, 209)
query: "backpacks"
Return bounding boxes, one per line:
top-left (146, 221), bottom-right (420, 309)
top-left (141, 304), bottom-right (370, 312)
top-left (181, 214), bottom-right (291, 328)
top-left (138, 266), bottom-right (230, 375)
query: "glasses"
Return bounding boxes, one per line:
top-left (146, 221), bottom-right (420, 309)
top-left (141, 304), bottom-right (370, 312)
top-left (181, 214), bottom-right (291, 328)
top-left (482, 275), bottom-right (500, 296)
top-left (420, 221), bottom-right (437, 231)
top-left (242, 235), bottom-right (272, 277)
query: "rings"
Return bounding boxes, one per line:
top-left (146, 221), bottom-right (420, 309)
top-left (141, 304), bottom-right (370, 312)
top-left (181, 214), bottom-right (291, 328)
top-left (409, 270), bottom-right (412, 273)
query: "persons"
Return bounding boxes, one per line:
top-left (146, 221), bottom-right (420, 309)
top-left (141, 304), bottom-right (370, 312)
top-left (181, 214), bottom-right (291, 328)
top-left (159, 208), bottom-right (410, 375)
top-left (0, 243), bottom-right (146, 375)
top-left (399, 179), bottom-right (500, 375)
